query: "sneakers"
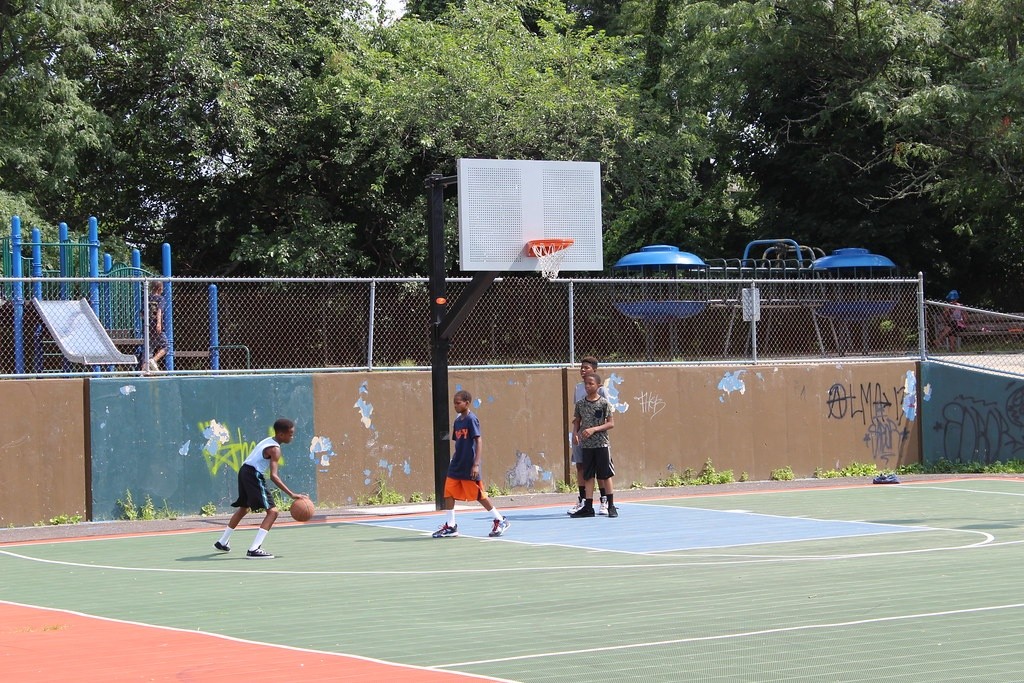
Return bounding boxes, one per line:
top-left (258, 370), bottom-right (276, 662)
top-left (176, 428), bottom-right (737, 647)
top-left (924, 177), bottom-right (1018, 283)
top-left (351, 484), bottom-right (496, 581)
top-left (566, 496), bottom-right (586, 515)
top-left (598, 496), bottom-right (608, 515)
top-left (608, 504), bottom-right (618, 517)
top-left (149, 359), bottom-right (159, 370)
top-left (432, 522), bottom-right (458, 538)
top-left (246, 545), bottom-right (274, 559)
top-left (570, 502), bottom-right (596, 518)
top-left (213, 541), bottom-right (232, 553)
top-left (489, 516), bottom-right (511, 537)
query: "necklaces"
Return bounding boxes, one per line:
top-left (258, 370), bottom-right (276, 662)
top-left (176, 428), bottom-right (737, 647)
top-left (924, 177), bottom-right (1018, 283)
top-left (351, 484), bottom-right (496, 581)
top-left (272, 437), bottom-right (281, 444)
top-left (468, 410), bottom-right (470, 415)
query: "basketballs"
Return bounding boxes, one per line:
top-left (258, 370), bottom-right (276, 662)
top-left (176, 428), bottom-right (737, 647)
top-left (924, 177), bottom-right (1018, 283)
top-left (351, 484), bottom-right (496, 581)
top-left (290, 498), bottom-right (315, 522)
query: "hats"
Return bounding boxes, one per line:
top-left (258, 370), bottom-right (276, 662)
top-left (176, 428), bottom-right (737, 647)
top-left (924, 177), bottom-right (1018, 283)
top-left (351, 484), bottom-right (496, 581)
top-left (946, 290), bottom-right (959, 300)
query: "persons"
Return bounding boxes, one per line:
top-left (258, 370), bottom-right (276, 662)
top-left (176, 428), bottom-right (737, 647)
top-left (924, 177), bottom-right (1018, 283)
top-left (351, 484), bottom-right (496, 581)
top-left (566, 358), bottom-right (619, 518)
top-left (140, 280), bottom-right (171, 370)
top-left (432, 390), bottom-right (511, 538)
top-left (214, 418), bottom-right (308, 558)
top-left (933, 291), bottom-right (967, 352)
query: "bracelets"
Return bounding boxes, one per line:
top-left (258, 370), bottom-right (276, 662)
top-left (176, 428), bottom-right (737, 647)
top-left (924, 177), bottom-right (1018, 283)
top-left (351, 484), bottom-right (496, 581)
top-left (473, 464), bottom-right (479, 466)
